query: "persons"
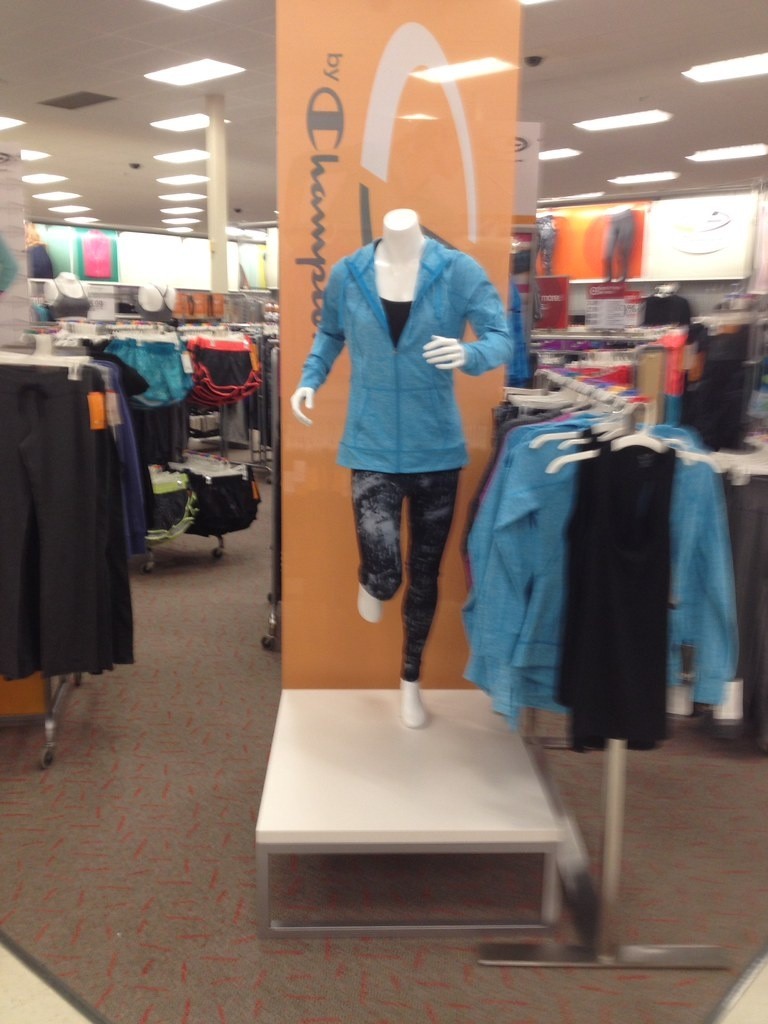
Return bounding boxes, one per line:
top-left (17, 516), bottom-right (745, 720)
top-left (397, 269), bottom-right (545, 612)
top-left (534, 213), bottom-right (556, 276)
top-left (44, 272), bottom-right (91, 319)
top-left (599, 204), bottom-right (633, 284)
top-left (290, 209), bottom-right (511, 728)
top-left (138, 281), bottom-right (176, 320)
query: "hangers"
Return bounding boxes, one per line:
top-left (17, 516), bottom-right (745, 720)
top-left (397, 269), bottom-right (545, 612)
top-left (149, 453), bottom-right (247, 488)
top-left (0, 322), bottom-right (249, 381)
top-left (507, 341), bottom-right (722, 475)
